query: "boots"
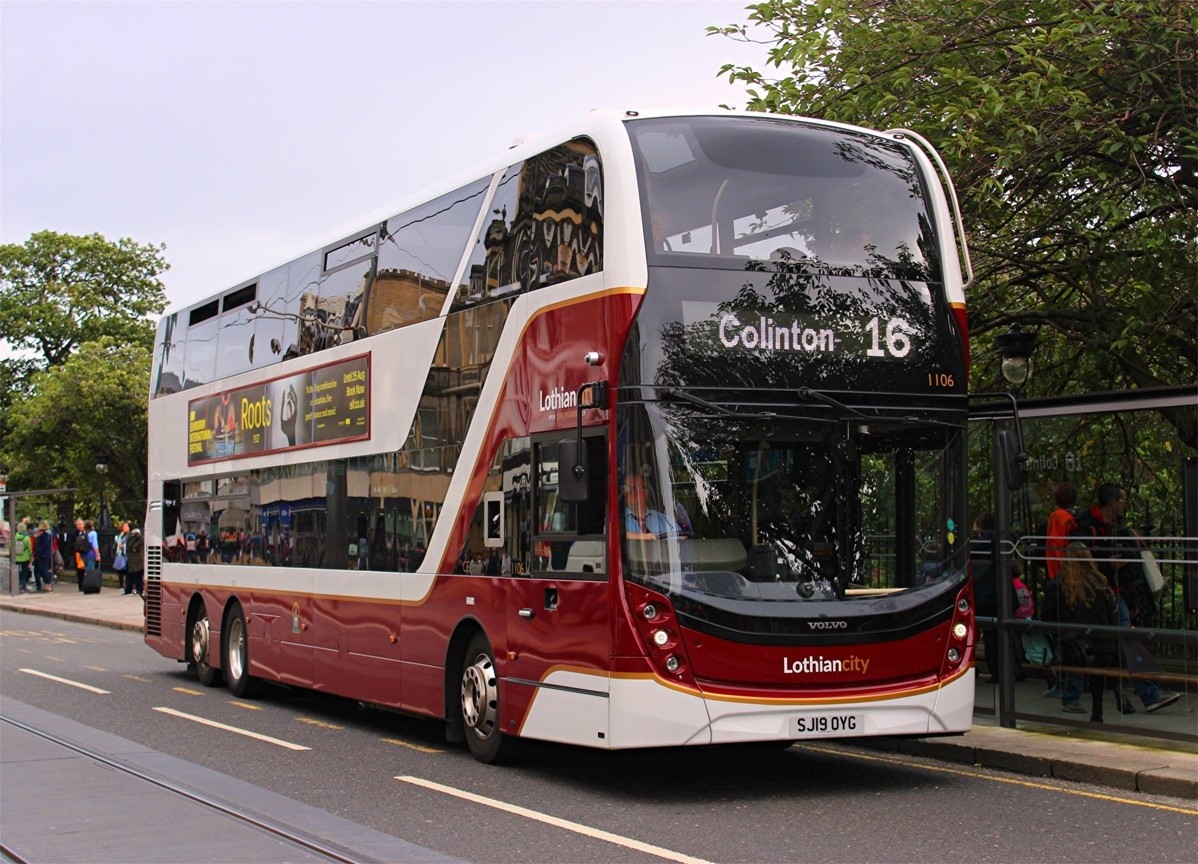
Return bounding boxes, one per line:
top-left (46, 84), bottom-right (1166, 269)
top-left (1113, 685), bottom-right (1137, 715)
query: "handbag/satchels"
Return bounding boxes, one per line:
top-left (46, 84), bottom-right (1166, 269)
top-left (1129, 528), bottom-right (1164, 593)
top-left (1021, 618), bottom-right (1053, 666)
top-left (54, 549), bottom-right (64, 566)
top-left (76, 552), bottom-right (86, 570)
top-left (112, 556), bottom-right (129, 571)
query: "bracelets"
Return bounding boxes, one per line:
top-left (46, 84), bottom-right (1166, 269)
top-left (95, 554), bottom-right (100, 556)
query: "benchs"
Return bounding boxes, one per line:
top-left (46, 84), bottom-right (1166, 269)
top-left (1029, 661), bottom-right (1196, 693)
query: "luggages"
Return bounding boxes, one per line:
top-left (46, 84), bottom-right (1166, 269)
top-left (82, 560), bottom-right (102, 594)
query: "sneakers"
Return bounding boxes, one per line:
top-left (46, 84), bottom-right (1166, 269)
top-left (1146, 693), bottom-right (1181, 712)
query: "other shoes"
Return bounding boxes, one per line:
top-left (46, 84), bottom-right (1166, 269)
top-left (42, 585), bottom-right (52, 592)
top-left (1062, 702), bottom-right (1087, 712)
top-left (122, 591), bottom-right (133, 598)
top-left (986, 673), bottom-right (999, 684)
top-left (1043, 685), bottom-right (1063, 697)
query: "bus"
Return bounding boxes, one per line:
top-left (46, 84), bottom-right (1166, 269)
top-left (137, 103), bottom-right (1036, 764)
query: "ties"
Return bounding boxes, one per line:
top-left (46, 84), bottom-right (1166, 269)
top-left (630, 513), bottom-right (651, 534)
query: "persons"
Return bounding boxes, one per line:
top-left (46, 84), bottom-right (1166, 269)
top-left (74, 518), bottom-right (101, 592)
top-left (650, 206), bottom-right (666, 252)
top-left (113, 522), bottom-right (144, 596)
top-left (9, 518), bottom-right (57, 593)
top-left (919, 481), bottom-right (1180, 715)
top-left (456, 546), bottom-right (524, 576)
top-left (626, 471), bottom-right (688, 541)
top-left (162, 526), bottom-right (427, 572)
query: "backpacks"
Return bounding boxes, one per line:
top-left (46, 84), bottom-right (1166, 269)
top-left (78, 531), bottom-right (91, 553)
top-left (15, 535), bottom-right (27, 554)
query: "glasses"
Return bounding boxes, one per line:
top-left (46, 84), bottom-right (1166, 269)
top-left (1112, 497), bottom-right (1131, 506)
top-left (621, 485), bottom-right (649, 493)
